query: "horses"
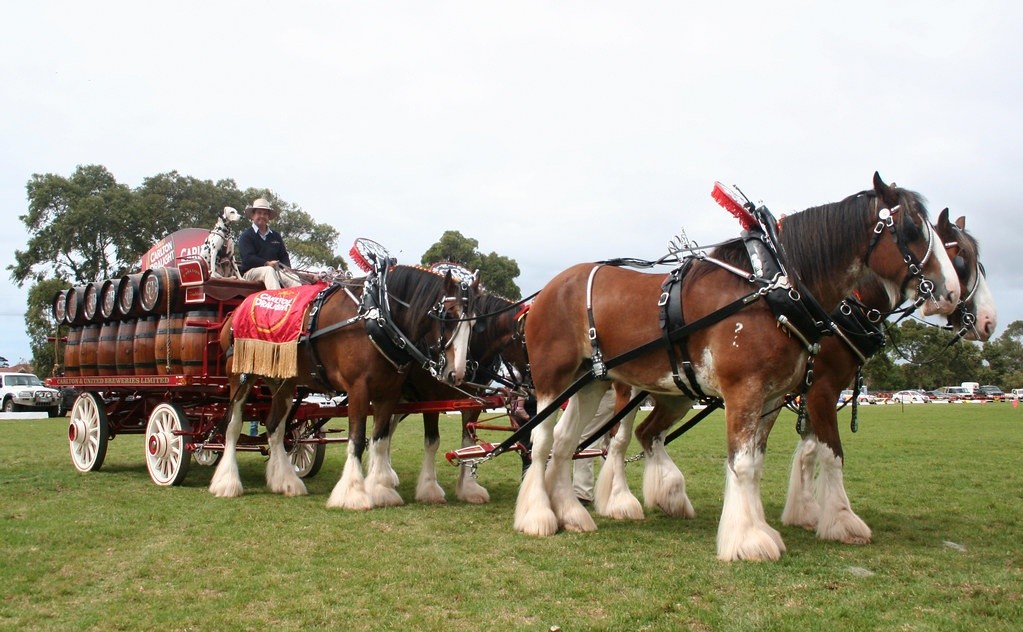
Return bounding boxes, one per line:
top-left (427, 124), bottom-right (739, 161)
top-left (206, 169), bottom-right (997, 563)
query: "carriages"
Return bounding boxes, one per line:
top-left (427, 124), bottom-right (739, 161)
top-left (40, 168), bottom-right (1002, 566)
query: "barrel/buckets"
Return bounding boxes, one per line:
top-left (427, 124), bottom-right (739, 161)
top-left (52, 266), bottom-right (232, 377)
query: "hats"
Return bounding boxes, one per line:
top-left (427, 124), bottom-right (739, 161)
top-left (245, 199), bottom-right (277, 220)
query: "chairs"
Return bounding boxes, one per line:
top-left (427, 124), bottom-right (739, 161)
top-left (17, 379), bottom-right (26, 385)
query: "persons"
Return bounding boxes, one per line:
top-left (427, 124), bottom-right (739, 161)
top-left (250, 421), bottom-right (258, 436)
top-left (239, 199), bottom-right (301, 290)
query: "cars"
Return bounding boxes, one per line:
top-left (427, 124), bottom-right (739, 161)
top-left (891, 390), bottom-right (930, 404)
top-left (41, 380), bottom-right (135, 417)
top-left (0, 372), bottom-right (62, 418)
top-left (925, 385), bottom-right (1006, 399)
top-left (292, 392), bottom-right (373, 408)
top-left (840, 388), bottom-right (876, 404)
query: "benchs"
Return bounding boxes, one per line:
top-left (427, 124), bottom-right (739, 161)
top-left (176, 254), bottom-right (265, 310)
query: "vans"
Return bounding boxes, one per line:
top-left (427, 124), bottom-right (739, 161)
top-left (1010, 387), bottom-right (1023, 401)
